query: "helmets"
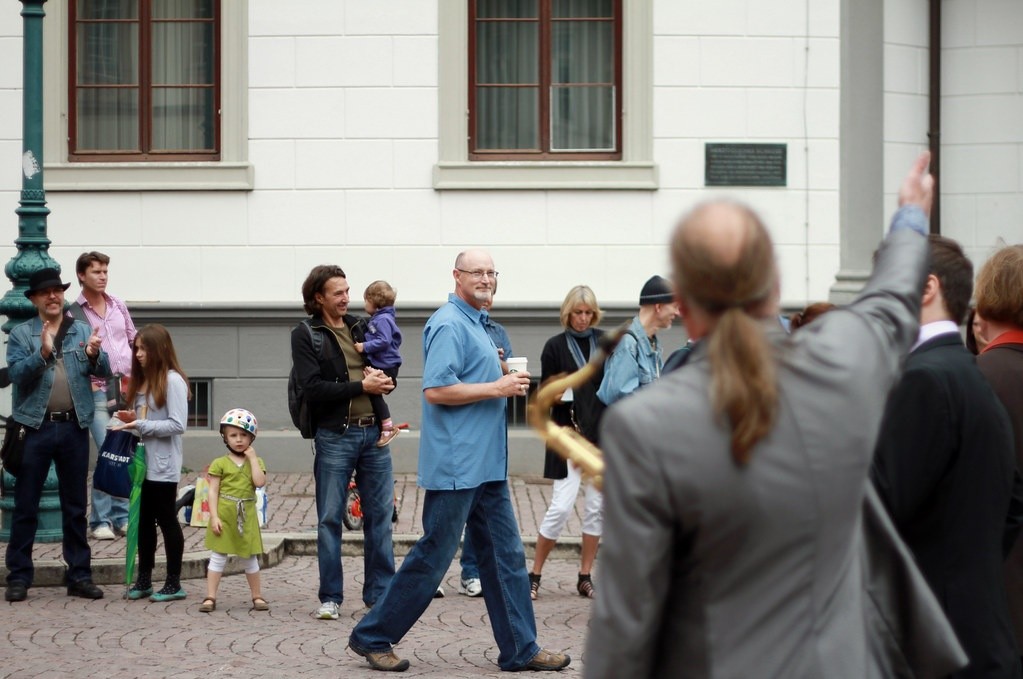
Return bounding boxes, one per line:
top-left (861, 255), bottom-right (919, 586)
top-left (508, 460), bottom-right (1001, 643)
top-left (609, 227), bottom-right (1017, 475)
top-left (218, 408), bottom-right (257, 437)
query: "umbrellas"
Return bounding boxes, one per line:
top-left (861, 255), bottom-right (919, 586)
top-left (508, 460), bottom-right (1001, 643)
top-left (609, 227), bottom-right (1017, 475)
top-left (124, 427), bottom-right (146, 603)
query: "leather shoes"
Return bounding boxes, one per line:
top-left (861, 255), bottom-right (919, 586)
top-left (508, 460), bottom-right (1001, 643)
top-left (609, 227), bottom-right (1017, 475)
top-left (5, 582), bottom-right (28, 602)
top-left (67, 580), bottom-right (103, 598)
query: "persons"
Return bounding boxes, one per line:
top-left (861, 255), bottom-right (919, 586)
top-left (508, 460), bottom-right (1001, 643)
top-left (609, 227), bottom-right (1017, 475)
top-left (114, 324), bottom-right (188, 601)
top-left (420, 268), bottom-right (697, 598)
top-left (288, 264), bottom-right (402, 619)
top-left (581, 152), bottom-right (942, 679)
top-left (4, 269), bottom-right (112, 601)
top-left (200, 408), bottom-right (269, 612)
top-left (348, 249), bottom-right (572, 673)
top-left (789, 234), bottom-right (1023, 679)
top-left (67, 252), bottom-right (139, 540)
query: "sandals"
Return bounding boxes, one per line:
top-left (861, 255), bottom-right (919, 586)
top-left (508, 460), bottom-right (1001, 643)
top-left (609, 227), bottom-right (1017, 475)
top-left (577, 573), bottom-right (596, 598)
top-left (526, 572), bottom-right (541, 600)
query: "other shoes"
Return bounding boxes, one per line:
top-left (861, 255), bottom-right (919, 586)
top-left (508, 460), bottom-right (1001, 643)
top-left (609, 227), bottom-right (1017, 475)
top-left (124, 585), bottom-right (153, 599)
top-left (118, 524), bottom-right (127, 534)
top-left (90, 526), bottom-right (115, 540)
top-left (252, 597), bottom-right (268, 611)
top-left (377, 426), bottom-right (400, 446)
top-left (198, 597), bottom-right (216, 611)
top-left (150, 588), bottom-right (185, 602)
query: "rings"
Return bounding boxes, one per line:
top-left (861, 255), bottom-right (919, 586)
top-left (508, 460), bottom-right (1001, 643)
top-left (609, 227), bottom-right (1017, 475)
top-left (520, 384), bottom-right (524, 390)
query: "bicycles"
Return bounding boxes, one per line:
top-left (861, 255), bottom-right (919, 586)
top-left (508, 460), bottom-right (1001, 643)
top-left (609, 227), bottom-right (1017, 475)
top-left (343, 422), bottom-right (410, 530)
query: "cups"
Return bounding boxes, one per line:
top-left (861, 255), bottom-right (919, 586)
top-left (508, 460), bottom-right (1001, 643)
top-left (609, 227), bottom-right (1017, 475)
top-left (506, 357), bottom-right (528, 375)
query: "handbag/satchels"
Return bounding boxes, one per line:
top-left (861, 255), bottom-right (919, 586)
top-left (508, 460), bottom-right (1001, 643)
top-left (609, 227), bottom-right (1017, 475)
top-left (191, 478), bottom-right (267, 526)
top-left (107, 371), bottom-right (132, 414)
top-left (93, 429), bottom-right (140, 498)
top-left (0, 415), bottom-right (28, 478)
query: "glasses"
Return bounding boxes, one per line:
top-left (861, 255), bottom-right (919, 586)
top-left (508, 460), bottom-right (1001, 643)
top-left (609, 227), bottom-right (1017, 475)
top-left (458, 270), bottom-right (500, 279)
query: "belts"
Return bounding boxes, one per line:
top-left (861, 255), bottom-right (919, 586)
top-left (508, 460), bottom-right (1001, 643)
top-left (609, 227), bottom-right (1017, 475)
top-left (350, 417), bottom-right (377, 428)
top-left (43, 411), bottom-right (77, 422)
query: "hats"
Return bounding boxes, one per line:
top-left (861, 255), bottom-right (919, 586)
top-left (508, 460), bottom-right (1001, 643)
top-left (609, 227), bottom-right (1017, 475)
top-left (23, 269), bottom-right (70, 299)
top-left (640, 275), bottom-right (678, 305)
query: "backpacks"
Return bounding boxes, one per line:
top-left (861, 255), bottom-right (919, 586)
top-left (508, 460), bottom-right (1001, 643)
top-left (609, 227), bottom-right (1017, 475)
top-left (288, 312), bottom-right (369, 440)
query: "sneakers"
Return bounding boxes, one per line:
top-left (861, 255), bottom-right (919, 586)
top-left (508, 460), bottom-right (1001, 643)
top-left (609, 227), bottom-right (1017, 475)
top-left (501, 648), bottom-right (571, 670)
top-left (435, 586), bottom-right (445, 598)
top-left (315, 602), bottom-right (339, 619)
top-left (347, 641), bottom-right (409, 671)
top-left (459, 578), bottom-right (483, 598)
